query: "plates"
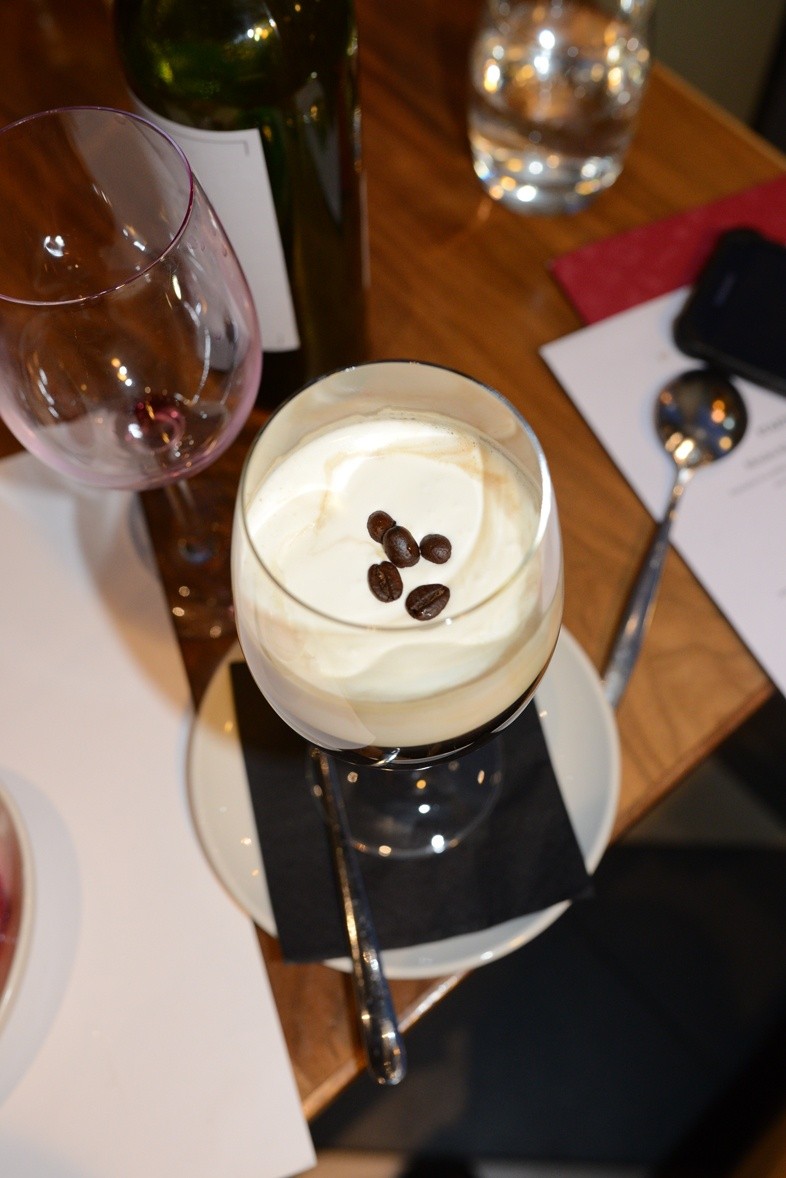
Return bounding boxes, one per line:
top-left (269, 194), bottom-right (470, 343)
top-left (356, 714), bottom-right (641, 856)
top-left (187, 621), bottom-right (622, 980)
top-left (0, 780), bottom-right (36, 1030)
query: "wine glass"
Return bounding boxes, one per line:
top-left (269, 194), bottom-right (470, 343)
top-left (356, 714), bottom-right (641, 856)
top-left (0, 105), bottom-right (262, 639)
top-left (468, 644), bottom-right (487, 658)
top-left (231, 360), bottom-right (566, 859)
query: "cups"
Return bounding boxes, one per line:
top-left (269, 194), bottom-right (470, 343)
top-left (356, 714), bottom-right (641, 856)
top-left (465, 0), bottom-right (659, 216)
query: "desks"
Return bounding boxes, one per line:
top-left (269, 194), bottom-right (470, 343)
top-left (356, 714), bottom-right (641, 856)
top-left (0, 0), bottom-right (786, 1123)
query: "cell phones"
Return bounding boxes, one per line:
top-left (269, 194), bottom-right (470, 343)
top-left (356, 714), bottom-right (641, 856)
top-left (671, 228), bottom-right (786, 400)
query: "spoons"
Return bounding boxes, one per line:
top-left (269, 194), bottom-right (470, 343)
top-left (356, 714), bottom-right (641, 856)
top-left (599, 369), bottom-right (749, 716)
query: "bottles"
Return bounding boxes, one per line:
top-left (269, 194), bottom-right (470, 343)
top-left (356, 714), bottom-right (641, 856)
top-left (110, 0), bottom-right (373, 445)
